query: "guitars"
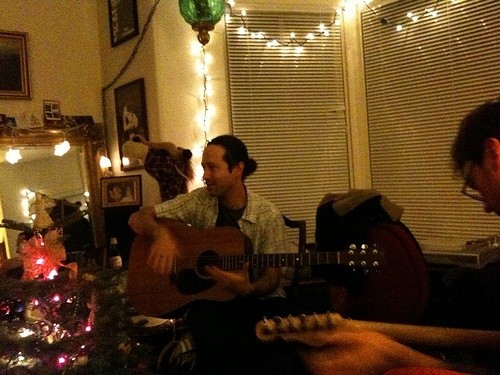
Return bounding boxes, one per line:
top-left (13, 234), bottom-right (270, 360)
top-left (124, 215), bottom-right (385, 322)
top-left (255, 309), bottom-right (500, 349)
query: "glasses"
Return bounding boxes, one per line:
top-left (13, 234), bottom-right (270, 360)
top-left (461, 152), bottom-right (486, 202)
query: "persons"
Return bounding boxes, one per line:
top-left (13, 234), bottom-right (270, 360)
top-left (110, 187), bottom-right (132, 201)
top-left (128, 135), bottom-right (288, 375)
top-left (280, 101), bottom-right (500, 375)
top-left (124, 108), bottom-right (145, 142)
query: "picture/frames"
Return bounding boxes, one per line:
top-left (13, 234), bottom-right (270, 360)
top-left (108, 0), bottom-right (139, 48)
top-left (100, 174), bottom-right (143, 209)
top-left (113, 78), bottom-right (150, 171)
top-left (0, 30), bottom-right (33, 100)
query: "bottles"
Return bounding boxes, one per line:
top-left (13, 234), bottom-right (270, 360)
top-left (107, 237), bottom-right (122, 268)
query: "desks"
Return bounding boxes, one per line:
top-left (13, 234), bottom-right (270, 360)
top-left (293, 236), bottom-right (500, 270)
